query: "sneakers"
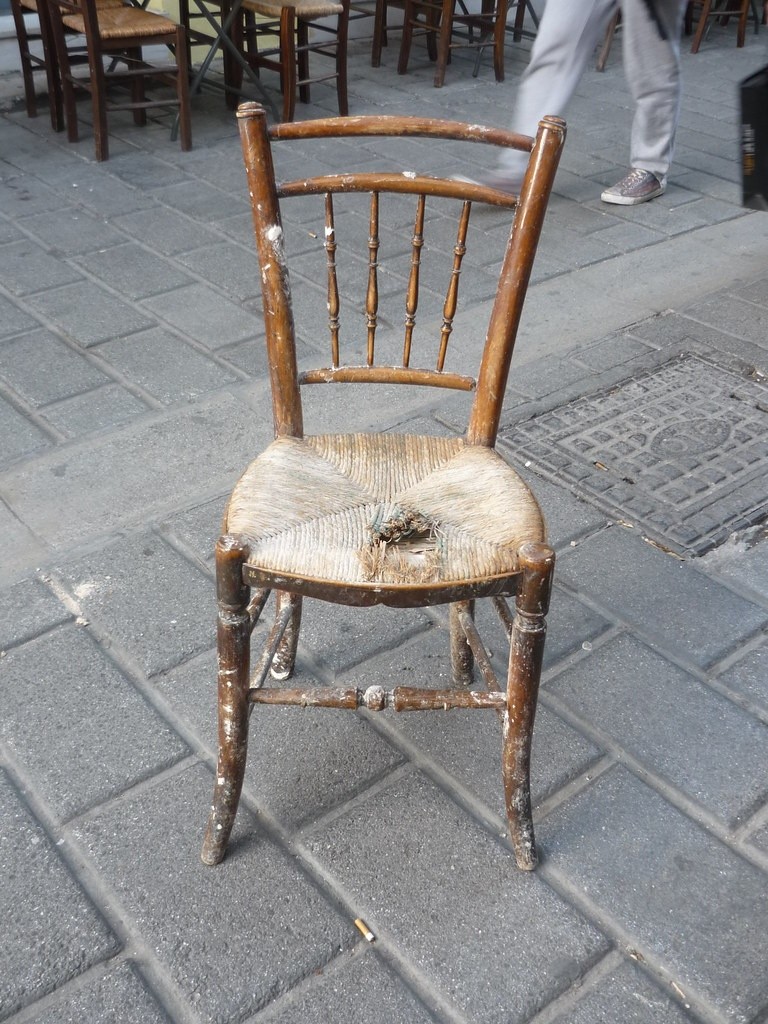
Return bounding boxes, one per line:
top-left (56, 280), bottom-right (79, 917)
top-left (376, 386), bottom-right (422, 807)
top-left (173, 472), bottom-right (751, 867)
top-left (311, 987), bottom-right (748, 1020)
top-left (600, 169), bottom-right (666, 205)
top-left (448, 172), bottom-right (523, 194)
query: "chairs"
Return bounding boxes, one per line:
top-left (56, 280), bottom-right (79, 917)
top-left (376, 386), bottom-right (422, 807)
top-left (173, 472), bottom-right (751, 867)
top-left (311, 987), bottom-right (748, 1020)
top-left (0, 0), bottom-right (768, 162)
top-left (200, 98), bottom-right (569, 873)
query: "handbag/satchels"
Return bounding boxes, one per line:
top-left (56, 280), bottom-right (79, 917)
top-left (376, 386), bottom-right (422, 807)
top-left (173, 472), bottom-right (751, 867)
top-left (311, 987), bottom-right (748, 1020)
top-left (739, 65), bottom-right (768, 213)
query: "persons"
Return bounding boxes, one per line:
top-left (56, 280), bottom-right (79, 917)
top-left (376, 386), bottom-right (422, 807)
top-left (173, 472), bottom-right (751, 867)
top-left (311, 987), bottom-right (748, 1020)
top-left (447, 1), bottom-right (690, 205)
